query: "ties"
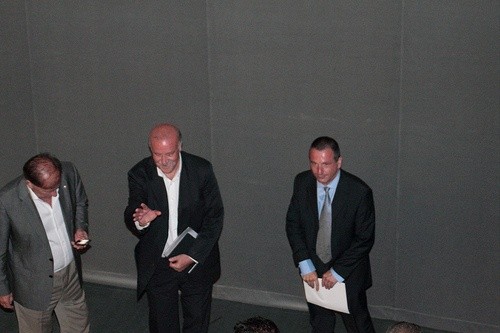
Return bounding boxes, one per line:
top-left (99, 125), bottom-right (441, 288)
top-left (315, 187), bottom-right (333, 264)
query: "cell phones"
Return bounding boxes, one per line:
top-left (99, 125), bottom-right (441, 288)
top-left (75, 239), bottom-right (92, 246)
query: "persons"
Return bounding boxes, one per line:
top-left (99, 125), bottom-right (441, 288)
top-left (0, 152), bottom-right (92, 333)
top-left (124, 124), bottom-right (224, 333)
top-left (285, 136), bottom-right (376, 333)
top-left (234, 316), bottom-right (280, 333)
top-left (386, 321), bottom-right (422, 333)
